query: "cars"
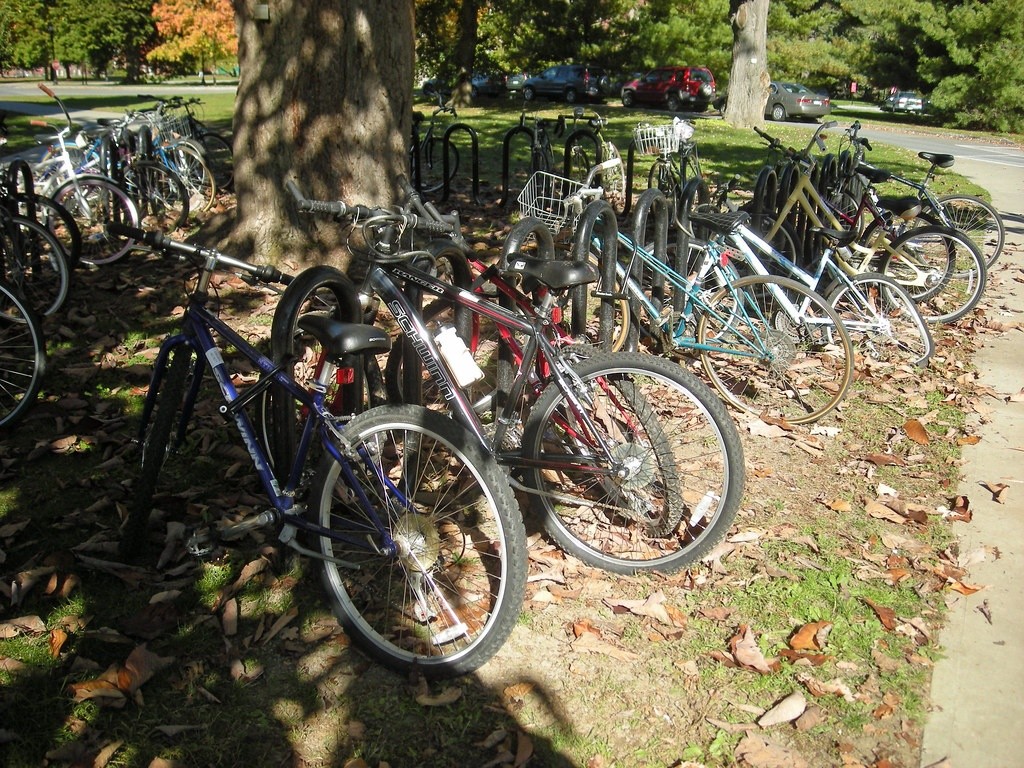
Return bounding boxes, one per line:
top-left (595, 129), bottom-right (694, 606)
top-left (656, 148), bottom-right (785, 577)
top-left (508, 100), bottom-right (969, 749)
top-left (884, 90), bottom-right (923, 115)
top-left (422, 69), bottom-right (507, 101)
top-left (714, 82), bottom-right (830, 121)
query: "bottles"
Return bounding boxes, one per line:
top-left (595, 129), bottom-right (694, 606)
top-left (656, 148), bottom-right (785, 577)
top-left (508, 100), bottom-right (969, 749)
top-left (433, 319), bottom-right (485, 389)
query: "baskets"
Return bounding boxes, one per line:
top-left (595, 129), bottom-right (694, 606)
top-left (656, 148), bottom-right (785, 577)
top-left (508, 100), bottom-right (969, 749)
top-left (632, 125), bottom-right (682, 155)
top-left (516, 170), bottom-right (585, 235)
top-left (156, 112), bottom-right (193, 143)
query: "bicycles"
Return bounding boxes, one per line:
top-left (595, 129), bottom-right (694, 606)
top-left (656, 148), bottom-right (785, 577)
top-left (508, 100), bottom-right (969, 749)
top-left (0, 80), bottom-right (236, 433)
top-left (104, 180), bottom-right (744, 679)
top-left (412, 92), bottom-right (458, 195)
top-left (513, 111), bottom-right (1008, 428)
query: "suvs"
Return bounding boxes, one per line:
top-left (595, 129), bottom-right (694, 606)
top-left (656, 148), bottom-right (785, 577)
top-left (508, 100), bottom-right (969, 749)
top-left (621, 66), bottom-right (715, 112)
top-left (520, 63), bottom-right (611, 106)
